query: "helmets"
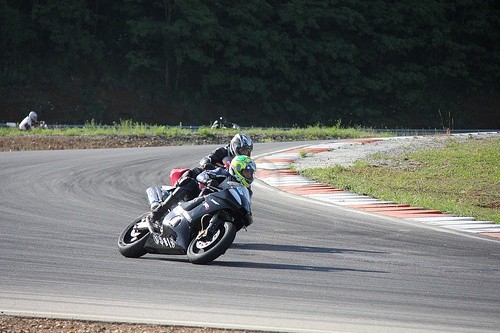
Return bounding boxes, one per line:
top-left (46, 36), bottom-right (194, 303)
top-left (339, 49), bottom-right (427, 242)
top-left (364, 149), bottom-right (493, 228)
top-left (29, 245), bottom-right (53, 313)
top-left (29, 111), bottom-right (37, 121)
top-left (229, 155), bottom-right (256, 187)
top-left (230, 134), bottom-right (253, 157)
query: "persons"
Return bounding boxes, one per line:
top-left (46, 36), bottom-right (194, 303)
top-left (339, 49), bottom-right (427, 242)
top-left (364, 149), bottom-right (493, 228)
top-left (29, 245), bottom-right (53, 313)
top-left (211, 116), bottom-right (227, 129)
top-left (162, 133), bottom-right (253, 202)
top-left (150, 155), bottom-right (256, 230)
top-left (18, 111), bottom-right (41, 131)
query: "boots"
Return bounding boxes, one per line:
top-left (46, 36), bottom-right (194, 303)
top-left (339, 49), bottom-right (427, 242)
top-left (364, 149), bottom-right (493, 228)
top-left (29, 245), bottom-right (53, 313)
top-left (151, 187), bottom-right (187, 221)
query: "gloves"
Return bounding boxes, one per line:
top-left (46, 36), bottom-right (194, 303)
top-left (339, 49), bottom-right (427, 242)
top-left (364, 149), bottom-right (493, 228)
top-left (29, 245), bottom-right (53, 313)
top-left (207, 180), bottom-right (219, 192)
top-left (205, 163), bottom-right (215, 170)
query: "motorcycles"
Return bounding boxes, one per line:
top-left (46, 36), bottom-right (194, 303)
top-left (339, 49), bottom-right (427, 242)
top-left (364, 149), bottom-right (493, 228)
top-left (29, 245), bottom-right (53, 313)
top-left (7, 121), bottom-right (48, 130)
top-left (212, 120), bottom-right (238, 130)
top-left (117, 168), bottom-right (253, 264)
